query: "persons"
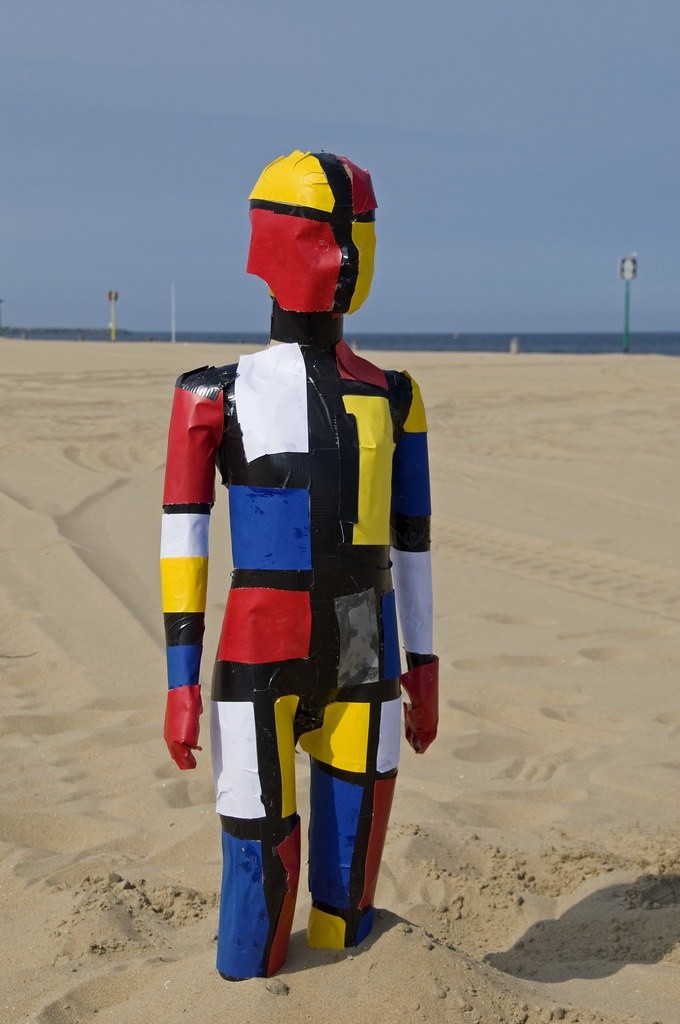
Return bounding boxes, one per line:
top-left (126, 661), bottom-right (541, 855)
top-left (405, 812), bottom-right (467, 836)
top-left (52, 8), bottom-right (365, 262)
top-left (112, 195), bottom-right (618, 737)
top-left (156, 151), bottom-right (442, 986)
